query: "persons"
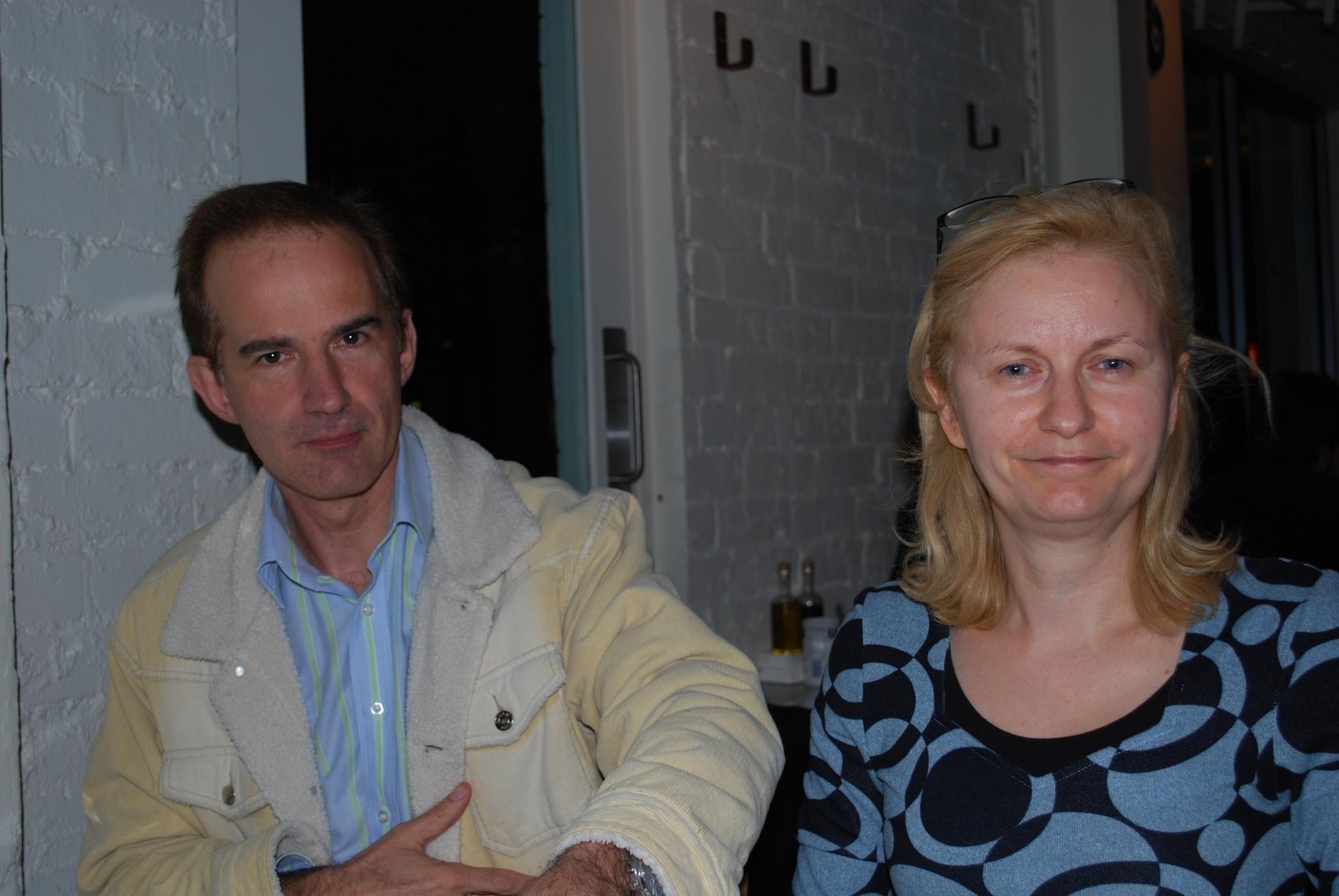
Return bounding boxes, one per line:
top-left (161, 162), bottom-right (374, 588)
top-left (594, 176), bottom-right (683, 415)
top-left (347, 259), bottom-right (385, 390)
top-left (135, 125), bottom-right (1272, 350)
top-left (788, 178), bottom-right (1339, 896)
top-left (77, 181), bottom-right (785, 896)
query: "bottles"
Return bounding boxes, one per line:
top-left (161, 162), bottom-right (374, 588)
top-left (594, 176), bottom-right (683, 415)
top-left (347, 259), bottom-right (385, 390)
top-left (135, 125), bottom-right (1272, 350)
top-left (770, 561), bottom-right (802, 655)
top-left (798, 560), bottom-right (826, 620)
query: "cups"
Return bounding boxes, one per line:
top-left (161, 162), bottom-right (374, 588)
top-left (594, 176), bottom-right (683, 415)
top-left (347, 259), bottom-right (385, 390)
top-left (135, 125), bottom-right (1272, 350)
top-left (805, 616), bottom-right (838, 688)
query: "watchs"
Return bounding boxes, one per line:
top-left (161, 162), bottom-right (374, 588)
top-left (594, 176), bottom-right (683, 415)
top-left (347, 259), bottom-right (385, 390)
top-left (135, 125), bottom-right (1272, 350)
top-left (624, 848), bottom-right (664, 896)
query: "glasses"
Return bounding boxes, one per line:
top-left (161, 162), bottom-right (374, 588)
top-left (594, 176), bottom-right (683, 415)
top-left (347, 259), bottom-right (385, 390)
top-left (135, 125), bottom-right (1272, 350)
top-left (936, 178), bottom-right (1136, 261)
